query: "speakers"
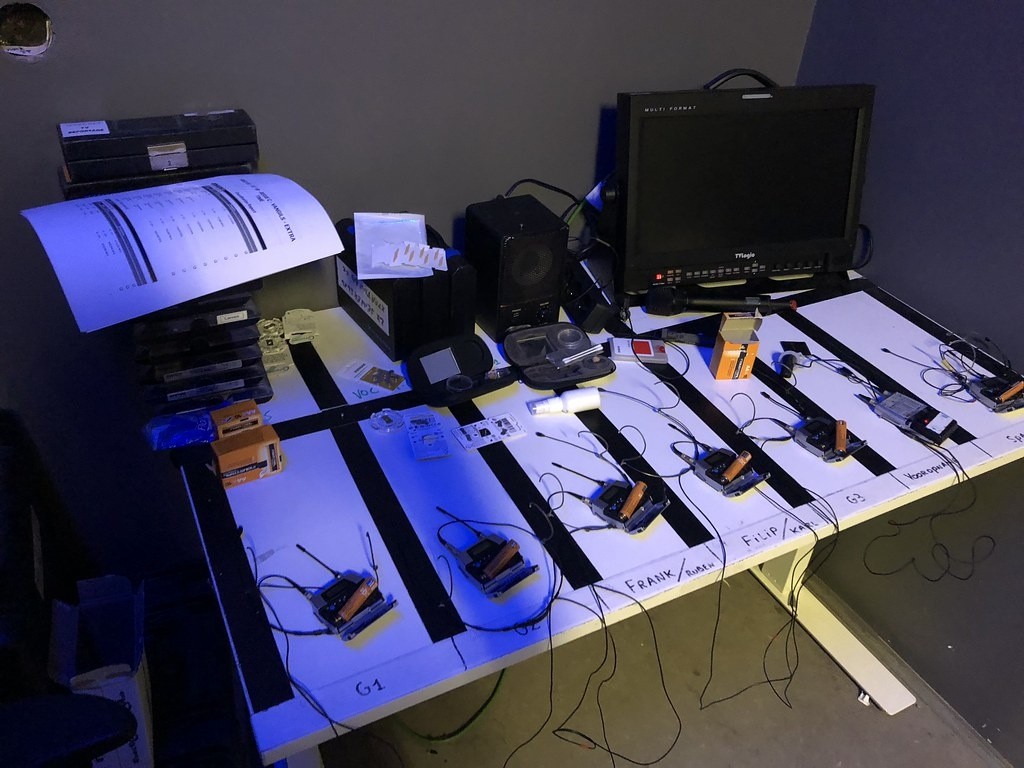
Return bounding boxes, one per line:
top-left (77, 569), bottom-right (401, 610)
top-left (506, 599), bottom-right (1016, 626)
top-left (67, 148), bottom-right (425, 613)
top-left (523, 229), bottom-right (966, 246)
top-left (466, 195), bottom-right (570, 343)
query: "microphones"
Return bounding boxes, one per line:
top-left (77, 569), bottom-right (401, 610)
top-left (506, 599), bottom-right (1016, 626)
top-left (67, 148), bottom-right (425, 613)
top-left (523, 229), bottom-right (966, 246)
top-left (644, 288), bottom-right (798, 316)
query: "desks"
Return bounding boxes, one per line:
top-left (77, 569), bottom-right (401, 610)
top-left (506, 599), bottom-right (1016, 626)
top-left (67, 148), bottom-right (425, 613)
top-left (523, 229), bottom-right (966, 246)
top-left (136, 270), bottom-right (1024, 768)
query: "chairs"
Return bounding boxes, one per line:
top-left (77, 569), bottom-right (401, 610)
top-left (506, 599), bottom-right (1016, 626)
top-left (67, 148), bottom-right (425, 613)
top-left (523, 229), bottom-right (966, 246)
top-left (0, 610), bottom-right (140, 768)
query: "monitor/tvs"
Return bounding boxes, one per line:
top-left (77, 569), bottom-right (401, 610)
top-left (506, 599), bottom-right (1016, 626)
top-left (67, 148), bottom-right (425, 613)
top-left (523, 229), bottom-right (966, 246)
top-left (615, 83), bottom-right (877, 295)
